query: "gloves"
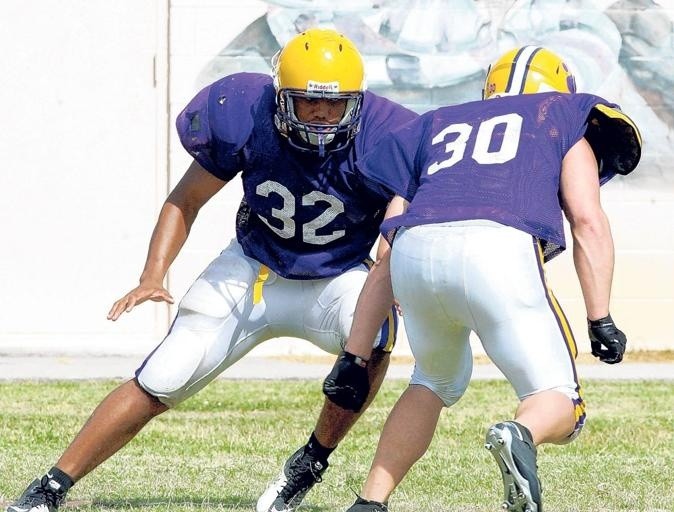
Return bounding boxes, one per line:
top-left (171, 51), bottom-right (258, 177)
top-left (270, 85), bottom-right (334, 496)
top-left (587, 312), bottom-right (627, 365)
top-left (322, 350), bottom-right (371, 413)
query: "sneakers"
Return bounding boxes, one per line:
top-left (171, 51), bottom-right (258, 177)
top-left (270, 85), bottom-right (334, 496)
top-left (485, 420), bottom-right (544, 512)
top-left (257, 444), bottom-right (329, 511)
top-left (7, 474), bottom-right (68, 512)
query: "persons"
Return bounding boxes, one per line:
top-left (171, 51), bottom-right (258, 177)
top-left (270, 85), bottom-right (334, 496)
top-left (6, 27), bottom-right (420, 512)
top-left (320, 45), bottom-right (645, 512)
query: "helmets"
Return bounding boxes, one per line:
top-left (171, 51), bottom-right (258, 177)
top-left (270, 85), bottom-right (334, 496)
top-left (482, 45), bottom-right (576, 100)
top-left (270, 27), bottom-right (364, 157)
